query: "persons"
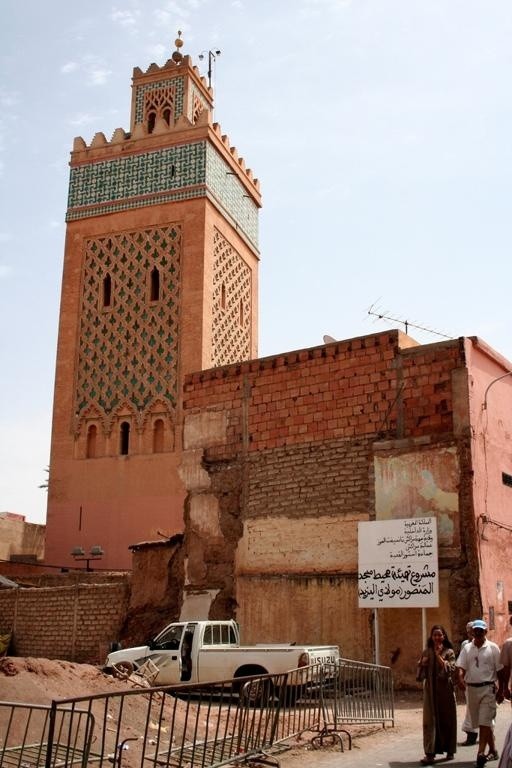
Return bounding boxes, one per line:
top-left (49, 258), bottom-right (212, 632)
top-left (500, 616), bottom-right (512, 700)
top-left (456, 620), bottom-right (503, 764)
top-left (414, 625), bottom-right (456, 763)
top-left (459, 621), bottom-right (496, 746)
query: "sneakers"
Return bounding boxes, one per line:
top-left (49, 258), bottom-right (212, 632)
top-left (416, 732), bottom-right (502, 768)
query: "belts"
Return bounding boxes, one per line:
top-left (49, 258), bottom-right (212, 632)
top-left (467, 682), bottom-right (493, 688)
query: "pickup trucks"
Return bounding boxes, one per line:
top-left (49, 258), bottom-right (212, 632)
top-left (106, 621), bottom-right (333, 693)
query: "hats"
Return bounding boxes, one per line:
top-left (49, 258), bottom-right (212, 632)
top-left (471, 619), bottom-right (488, 631)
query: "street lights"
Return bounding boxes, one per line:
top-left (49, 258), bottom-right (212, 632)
top-left (70, 546), bottom-right (104, 572)
